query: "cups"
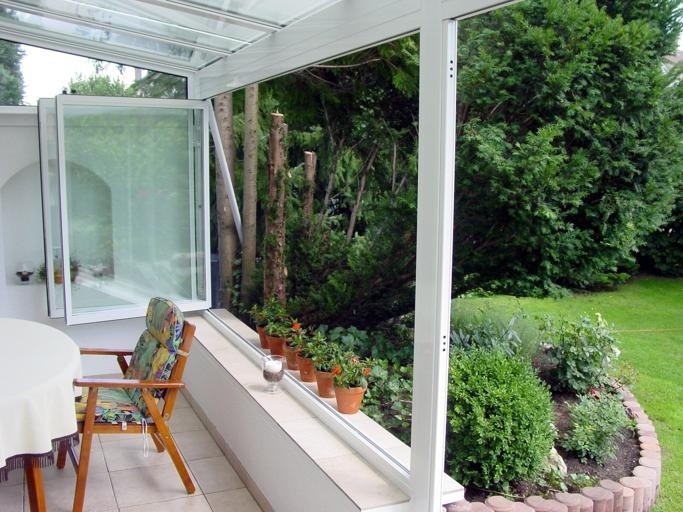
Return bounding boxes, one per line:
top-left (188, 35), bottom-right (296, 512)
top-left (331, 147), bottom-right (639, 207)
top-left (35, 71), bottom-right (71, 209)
top-left (16, 263), bottom-right (36, 285)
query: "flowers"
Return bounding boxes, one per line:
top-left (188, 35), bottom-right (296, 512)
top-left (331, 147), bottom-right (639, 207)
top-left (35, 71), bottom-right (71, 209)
top-left (330, 347), bottom-right (370, 391)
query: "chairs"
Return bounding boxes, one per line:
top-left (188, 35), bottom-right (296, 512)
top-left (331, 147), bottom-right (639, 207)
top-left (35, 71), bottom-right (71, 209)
top-left (55, 294), bottom-right (198, 512)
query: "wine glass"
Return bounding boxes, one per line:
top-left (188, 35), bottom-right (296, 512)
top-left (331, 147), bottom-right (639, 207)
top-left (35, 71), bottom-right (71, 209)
top-left (262, 354), bottom-right (288, 394)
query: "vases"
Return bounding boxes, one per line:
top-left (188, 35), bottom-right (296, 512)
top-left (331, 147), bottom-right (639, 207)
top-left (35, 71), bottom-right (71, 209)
top-left (334, 384), bottom-right (367, 414)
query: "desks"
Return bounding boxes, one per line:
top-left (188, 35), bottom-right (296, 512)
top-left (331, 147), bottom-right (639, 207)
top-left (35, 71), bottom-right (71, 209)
top-left (0, 316), bottom-right (84, 511)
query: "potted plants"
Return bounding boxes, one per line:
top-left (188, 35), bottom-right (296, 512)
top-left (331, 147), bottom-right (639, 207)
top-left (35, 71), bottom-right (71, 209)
top-left (248, 294), bottom-right (344, 398)
